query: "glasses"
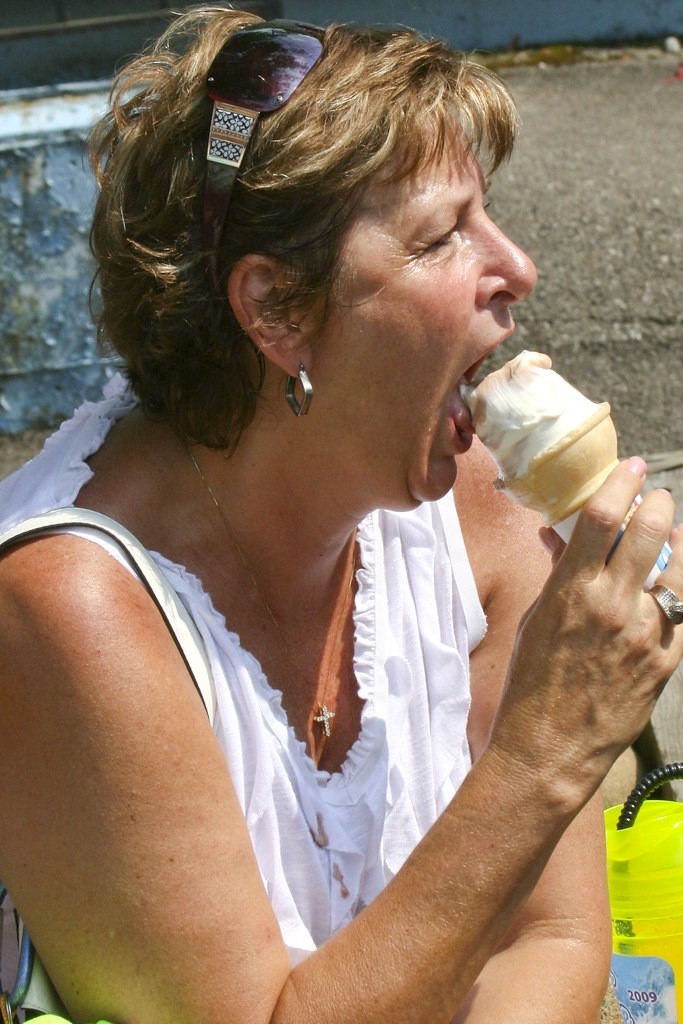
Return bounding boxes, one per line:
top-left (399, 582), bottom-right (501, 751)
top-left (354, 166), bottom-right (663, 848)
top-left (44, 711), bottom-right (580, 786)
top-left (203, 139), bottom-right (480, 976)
top-left (199, 18), bottom-right (326, 310)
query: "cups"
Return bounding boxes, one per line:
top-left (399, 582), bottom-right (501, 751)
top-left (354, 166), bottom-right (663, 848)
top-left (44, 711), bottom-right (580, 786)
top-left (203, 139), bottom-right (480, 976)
top-left (601, 800), bottom-right (682, 1024)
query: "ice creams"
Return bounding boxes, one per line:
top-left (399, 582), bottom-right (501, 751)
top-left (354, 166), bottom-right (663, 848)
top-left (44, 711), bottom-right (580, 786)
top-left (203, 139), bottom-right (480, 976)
top-left (458, 350), bottom-right (624, 524)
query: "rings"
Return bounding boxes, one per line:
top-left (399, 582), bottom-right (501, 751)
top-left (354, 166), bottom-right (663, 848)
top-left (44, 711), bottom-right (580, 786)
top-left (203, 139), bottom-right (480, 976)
top-left (649, 585), bottom-right (683, 625)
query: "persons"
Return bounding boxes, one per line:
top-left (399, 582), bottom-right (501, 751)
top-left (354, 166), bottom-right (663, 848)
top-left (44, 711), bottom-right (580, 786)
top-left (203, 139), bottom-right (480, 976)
top-left (0, 8), bottom-right (683, 1023)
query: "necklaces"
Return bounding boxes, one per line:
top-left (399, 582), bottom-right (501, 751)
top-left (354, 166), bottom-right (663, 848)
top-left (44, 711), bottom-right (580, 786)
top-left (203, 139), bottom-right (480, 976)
top-left (188, 428), bottom-right (356, 737)
